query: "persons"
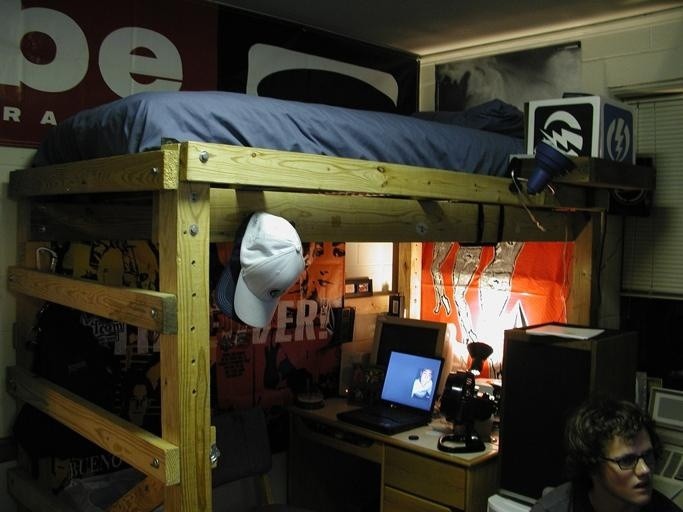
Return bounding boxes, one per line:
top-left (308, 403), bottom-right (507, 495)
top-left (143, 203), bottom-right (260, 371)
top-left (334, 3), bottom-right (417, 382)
top-left (428, 242), bottom-right (452, 316)
top-left (476, 242), bottom-right (525, 336)
top-left (447, 246), bottom-right (483, 342)
top-left (309, 241), bottom-right (345, 305)
top-left (520, 397), bottom-right (681, 510)
top-left (411, 366), bottom-right (434, 399)
top-left (279, 244), bottom-right (312, 308)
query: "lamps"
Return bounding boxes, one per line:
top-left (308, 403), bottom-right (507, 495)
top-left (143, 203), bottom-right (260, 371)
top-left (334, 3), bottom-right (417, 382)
top-left (467, 343), bottom-right (493, 375)
top-left (504, 138), bottom-right (578, 196)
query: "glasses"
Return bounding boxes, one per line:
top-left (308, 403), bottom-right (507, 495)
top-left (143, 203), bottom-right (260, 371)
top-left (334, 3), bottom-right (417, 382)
top-left (600, 450), bottom-right (657, 470)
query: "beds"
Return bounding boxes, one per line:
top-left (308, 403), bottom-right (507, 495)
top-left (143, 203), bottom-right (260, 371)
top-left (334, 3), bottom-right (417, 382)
top-left (6, 92), bottom-right (656, 512)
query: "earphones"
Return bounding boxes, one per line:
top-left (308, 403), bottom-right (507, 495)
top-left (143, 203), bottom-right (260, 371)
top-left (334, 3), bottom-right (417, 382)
top-left (490, 435), bottom-right (497, 442)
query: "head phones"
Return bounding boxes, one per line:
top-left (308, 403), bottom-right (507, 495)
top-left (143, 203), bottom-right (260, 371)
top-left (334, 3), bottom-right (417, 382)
top-left (437, 430), bottom-right (486, 453)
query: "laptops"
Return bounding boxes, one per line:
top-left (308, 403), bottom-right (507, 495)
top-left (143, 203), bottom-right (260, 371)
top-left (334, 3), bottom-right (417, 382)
top-left (336, 345), bottom-right (445, 435)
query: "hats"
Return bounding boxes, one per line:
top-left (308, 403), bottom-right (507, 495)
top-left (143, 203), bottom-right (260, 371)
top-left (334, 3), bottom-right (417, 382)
top-left (216, 210), bottom-right (304, 329)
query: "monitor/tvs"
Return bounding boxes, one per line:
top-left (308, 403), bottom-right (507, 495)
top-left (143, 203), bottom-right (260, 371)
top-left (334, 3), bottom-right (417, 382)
top-left (370, 318), bottom-right (448, 378)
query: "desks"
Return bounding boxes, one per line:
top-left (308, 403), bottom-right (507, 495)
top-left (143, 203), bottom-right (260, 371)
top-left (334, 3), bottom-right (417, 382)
top-left (294, 398), bottom-right (503, 512)
